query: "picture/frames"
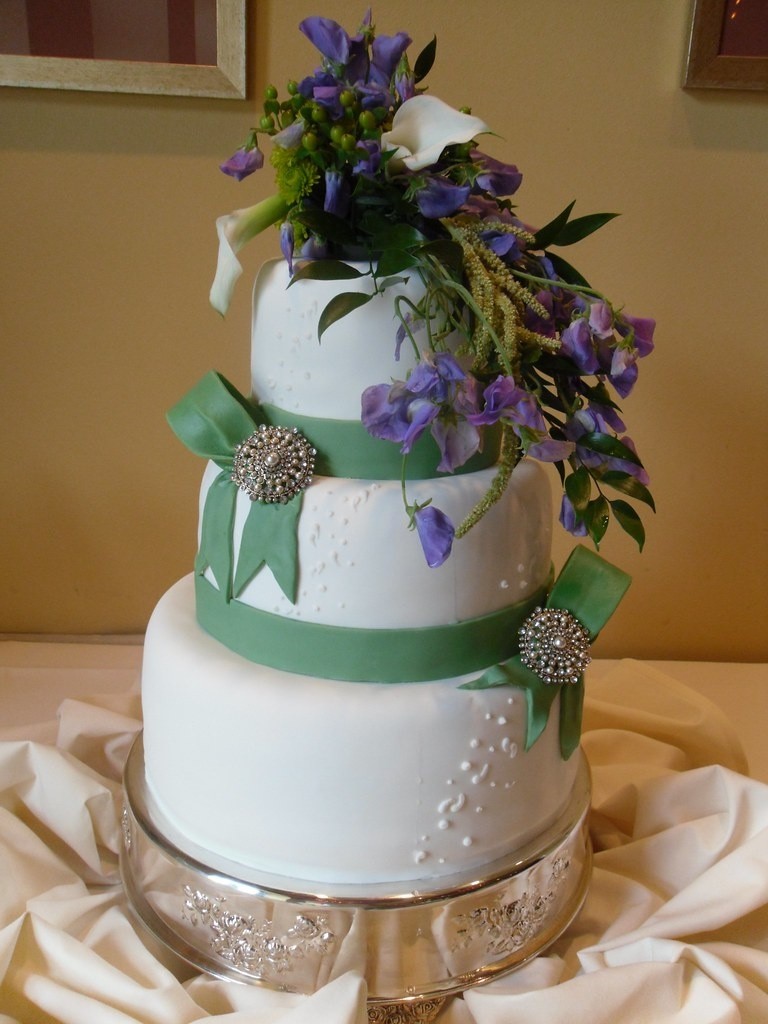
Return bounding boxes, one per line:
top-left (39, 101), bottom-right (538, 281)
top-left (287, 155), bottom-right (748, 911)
top-left (0, 0), bottom-right (247, 99)
top-left (680, 0), bottom-right (768, 93)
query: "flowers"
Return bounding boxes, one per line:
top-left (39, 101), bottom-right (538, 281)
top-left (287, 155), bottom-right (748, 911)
top-left (214, 9), bottom-right (664, 571)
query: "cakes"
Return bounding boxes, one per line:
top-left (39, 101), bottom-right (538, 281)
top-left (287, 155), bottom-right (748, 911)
top-left (139, 253), bottom-right (582, 886)
